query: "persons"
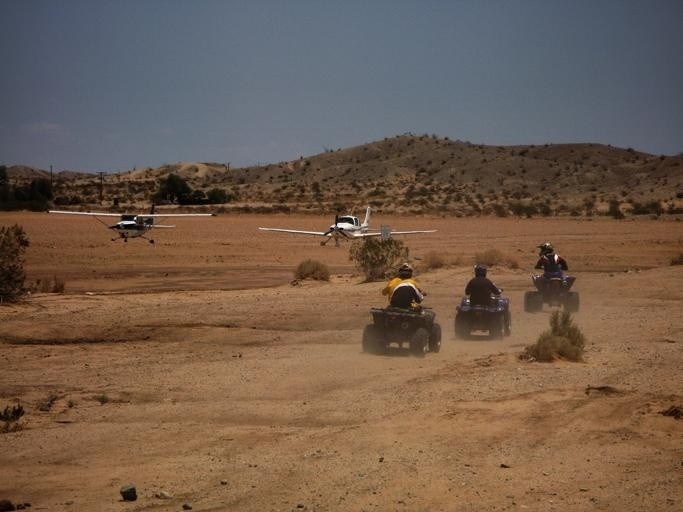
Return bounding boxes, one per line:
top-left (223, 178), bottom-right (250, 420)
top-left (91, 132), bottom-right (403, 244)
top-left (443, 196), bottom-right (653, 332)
top-left (534, 240), bottom-right (570, 291)
top-left (464, 264), bottom-right (503, 307)
top-left (380, 262), bottom-right (428, 314)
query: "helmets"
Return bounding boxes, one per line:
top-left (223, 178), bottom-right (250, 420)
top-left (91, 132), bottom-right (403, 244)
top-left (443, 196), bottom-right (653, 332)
top-left (540, 243), bottom-right (554, 254)
top-left (398, 263), bottom-right (413, 278)
top-left (474, 263), bottom-right (487, 276)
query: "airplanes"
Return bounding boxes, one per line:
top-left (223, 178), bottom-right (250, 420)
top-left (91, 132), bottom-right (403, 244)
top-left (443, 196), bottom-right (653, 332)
top-left (259, 206), bottom-right (438, 247)
top-left (46, 203), bottom-right (218, 244)
